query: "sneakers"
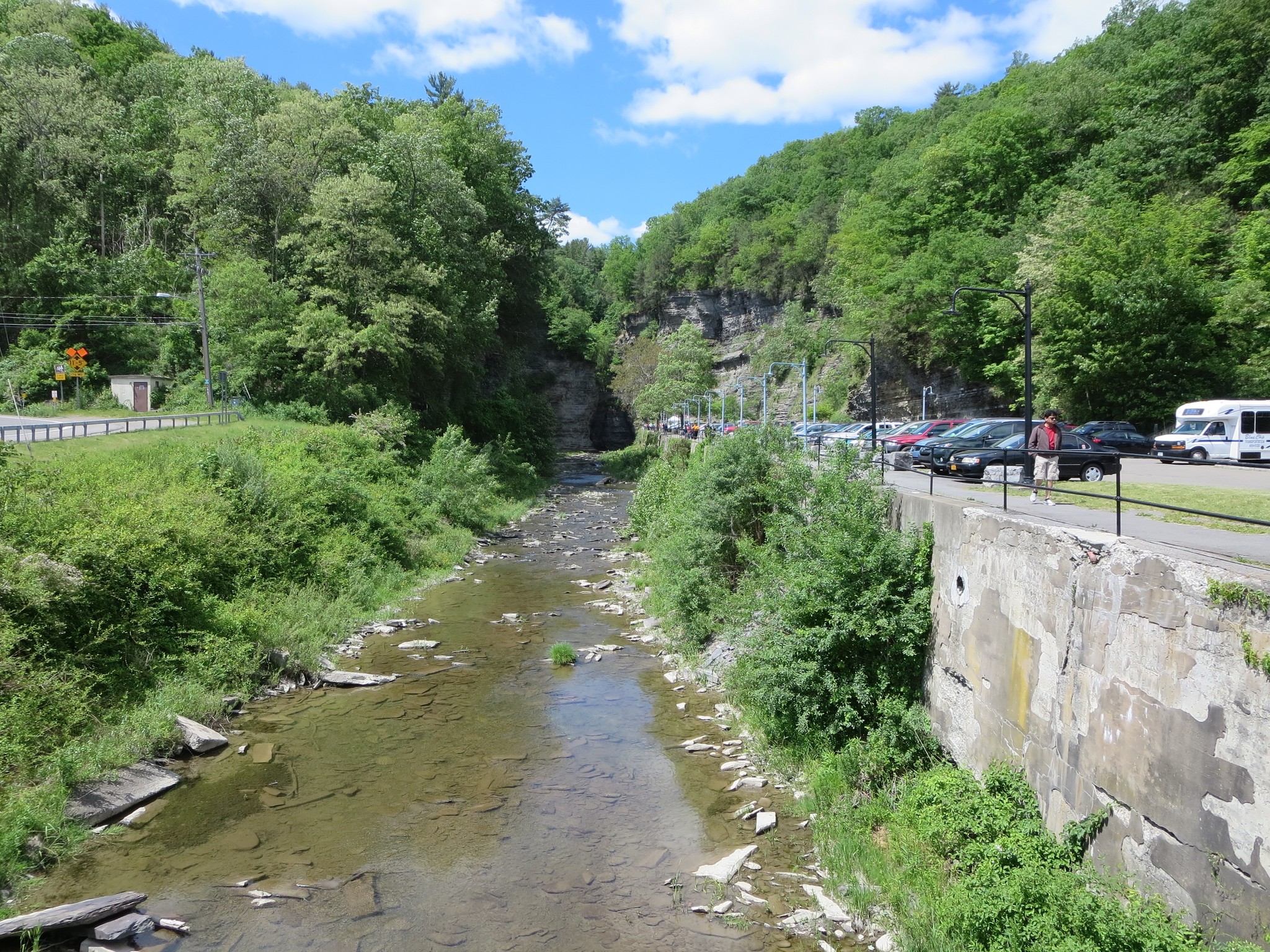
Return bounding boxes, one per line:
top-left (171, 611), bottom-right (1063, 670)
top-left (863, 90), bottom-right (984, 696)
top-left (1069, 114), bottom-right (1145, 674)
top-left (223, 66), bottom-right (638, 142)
top-left (1030, 489), bottom-right (1038, 502)
top-left (1044, 499), bottom-right (1055, 506)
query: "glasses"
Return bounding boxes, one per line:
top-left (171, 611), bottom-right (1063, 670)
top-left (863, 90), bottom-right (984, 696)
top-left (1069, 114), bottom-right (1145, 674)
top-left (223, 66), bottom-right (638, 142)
top-left (1047, 417), bottom-right (1057, 420)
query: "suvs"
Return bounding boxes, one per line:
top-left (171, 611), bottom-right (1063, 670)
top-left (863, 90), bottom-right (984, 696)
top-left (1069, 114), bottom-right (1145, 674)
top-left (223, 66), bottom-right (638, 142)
top-left (1069, 421), bottom-right (1138, 436)
top-left (784, 417), bottom-right (1069, 474)
top-left (723, 420), bottom-right (760, 435)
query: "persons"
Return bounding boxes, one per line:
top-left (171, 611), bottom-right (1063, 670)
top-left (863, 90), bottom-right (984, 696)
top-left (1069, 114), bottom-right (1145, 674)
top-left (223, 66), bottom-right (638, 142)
top-left (646, 423), bottom-right (649, 430)
top-left (1028, 410), bottom-right (1062, 506)
top-left (780, 423), bottom-right (783, 427)
top-left (662, 423), bottom-right (667, 436)
top-left (682, 423), bottom-right (699, 439)
top-left (704, 422), bottom-right (716, 442)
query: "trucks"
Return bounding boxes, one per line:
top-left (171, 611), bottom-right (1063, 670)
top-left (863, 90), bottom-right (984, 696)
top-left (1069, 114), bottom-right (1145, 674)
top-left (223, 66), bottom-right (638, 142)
top-left (1151, 400), bottom-right (1270, 465)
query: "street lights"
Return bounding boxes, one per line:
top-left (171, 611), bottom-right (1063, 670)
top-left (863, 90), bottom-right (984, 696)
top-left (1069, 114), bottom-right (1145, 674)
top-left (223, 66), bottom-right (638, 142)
top-left (156, 292), bottom-right (213, 406)
top-left (671, 401), bottom-right (691, 428)
top-left (735, 372), bottom-right (767, 426)
top-left (820, 331), bottom-right (877, 451)
top-left (692, 393), bottom-right (711, 427)
top-left (683, 397), bottom-right (701, 427)
top-left (940, 278), bottom-right (1035, 485)
top-left (923, 385), bottom-right (935, 420)
top-left (717, 381), bottom-right (743, 428)
top-left (765, 357), bottom-right (808, 449)
top-left (703, 388), bottom-right (726, 434)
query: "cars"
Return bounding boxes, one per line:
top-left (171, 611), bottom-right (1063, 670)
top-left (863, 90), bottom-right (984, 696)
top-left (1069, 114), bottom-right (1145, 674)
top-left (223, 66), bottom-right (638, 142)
top-left (667, 420), bottom-right (735, 433)
top-left (1084, 430), bottom-right (1154, 457)
top-left (947, 431), bottom-right (1122, 483)
